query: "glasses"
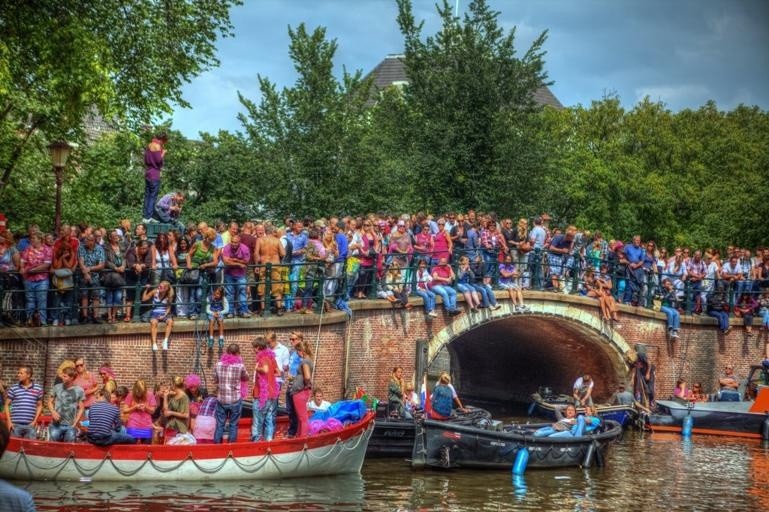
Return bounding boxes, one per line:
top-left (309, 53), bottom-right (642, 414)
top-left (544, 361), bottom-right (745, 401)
top-left (76, 365), bottom-right (83, 367)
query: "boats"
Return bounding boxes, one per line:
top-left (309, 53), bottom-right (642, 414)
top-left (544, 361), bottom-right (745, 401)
top-left (0, 394), bottom-right (377, 477)
top-left (406, 407), bottom-right (623, 472)
top-left (212, 392), bottom-right (497, 457)
top-left (526, 385), bottom-right (636, 432)
top-left (629, 360), bottom-right (769, 440)
top-left (1, 471), bottom-right (370, 512)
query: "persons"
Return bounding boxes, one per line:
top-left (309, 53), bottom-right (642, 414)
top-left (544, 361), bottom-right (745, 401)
top-left (0, 420), bottom-right (36, 512)
top-left (0, 202), bottom-right (767, 447)
top-left (141, 131), bottom-right (167, 224)
top-left (155, 191), bottom-right (186, 235)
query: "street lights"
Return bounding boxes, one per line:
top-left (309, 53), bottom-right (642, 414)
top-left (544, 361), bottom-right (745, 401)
top-left (45, 130), bottom-right (72, 240)
